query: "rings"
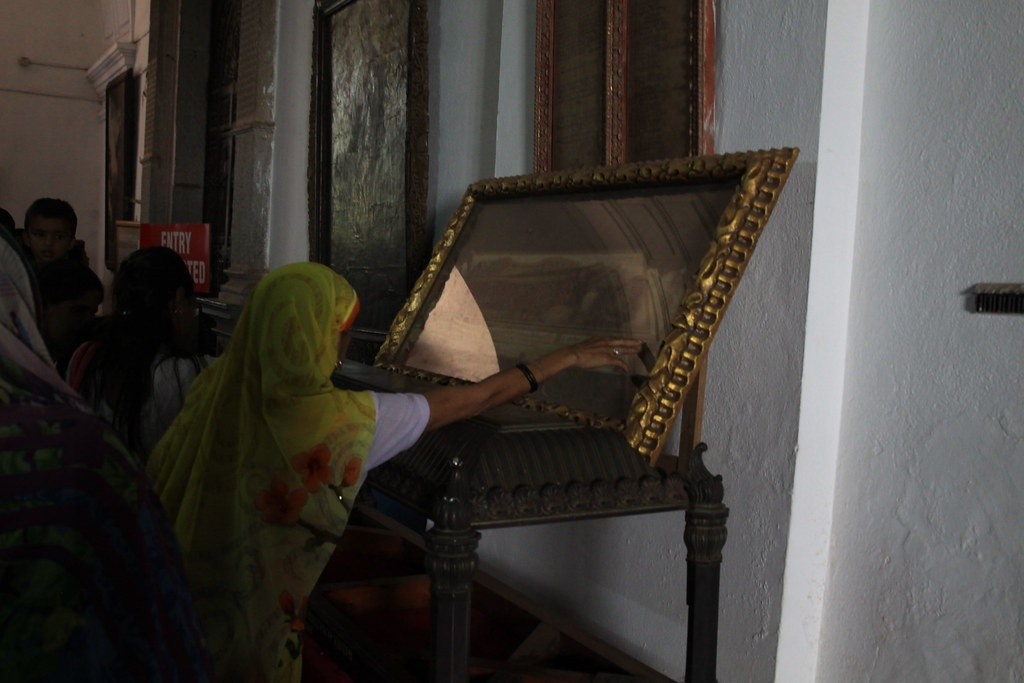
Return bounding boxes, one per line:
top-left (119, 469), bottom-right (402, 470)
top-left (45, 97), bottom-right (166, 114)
top-left (613, 348), bottom-right (619, 355)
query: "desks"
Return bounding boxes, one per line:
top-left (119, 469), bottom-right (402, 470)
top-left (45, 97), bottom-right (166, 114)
top-left (201, 310), bottom-right (728, 682)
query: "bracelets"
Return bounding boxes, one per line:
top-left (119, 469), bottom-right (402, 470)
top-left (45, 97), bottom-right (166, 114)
top-left (516, 363), bottom-right (540, 393)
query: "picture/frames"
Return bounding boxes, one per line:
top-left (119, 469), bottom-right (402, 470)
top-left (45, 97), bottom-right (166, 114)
top-left (376, 145), bottom-right (801, 464)
top-left (308, 0), bottom-right (431, 340)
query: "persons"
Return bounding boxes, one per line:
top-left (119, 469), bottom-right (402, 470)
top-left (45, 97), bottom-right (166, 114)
top-left (15, 196), bottom-right (105, 355)
top-left (67, 244), bottom-right (209, 452)
top-left (141, 263), bottom-right (640, 682)
